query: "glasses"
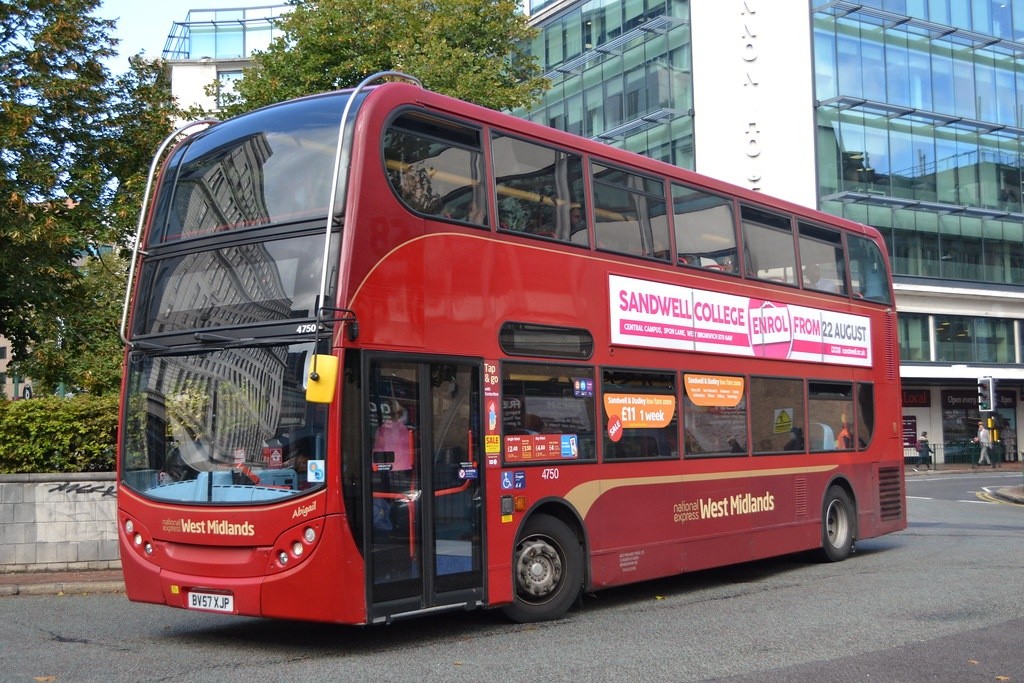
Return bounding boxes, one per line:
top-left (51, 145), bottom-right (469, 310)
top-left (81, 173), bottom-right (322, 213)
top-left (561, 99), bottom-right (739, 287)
top-left (723, 260), bottom-right (733, 265)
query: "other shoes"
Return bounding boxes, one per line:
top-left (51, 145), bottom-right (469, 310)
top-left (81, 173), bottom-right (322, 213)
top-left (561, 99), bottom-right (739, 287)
top-left (928, 469), bottom-right (934, 471)
top-left (913, 467), bottom-right (918, 472)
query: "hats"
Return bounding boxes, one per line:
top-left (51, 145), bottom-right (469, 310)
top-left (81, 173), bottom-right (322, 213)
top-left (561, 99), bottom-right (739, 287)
top-left (841, 414), bottom-right (847, 424)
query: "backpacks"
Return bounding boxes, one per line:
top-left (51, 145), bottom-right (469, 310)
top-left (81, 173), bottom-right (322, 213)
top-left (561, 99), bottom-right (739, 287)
top-left (914, 440), bottom-right (921, 452)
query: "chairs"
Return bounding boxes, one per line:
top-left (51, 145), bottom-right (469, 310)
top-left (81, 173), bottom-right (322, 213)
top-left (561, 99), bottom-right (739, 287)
top-left (442, 211), bottom-right (864, 298)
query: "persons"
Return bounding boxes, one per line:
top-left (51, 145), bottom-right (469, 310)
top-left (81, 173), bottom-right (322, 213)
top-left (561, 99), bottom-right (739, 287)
top-left (727, 437), bottom-right (743, 454)
top-left (913, 431), bottom-right (934, 472)
top-left (783, 427), bottom-right (804, 451)
top-left (720, 254), bottom-right (738, 274)
top-left (837, 421), bottom-right (852, 450)
top-left (1001, 424), bottom-right (1016, 462)
top-left (570, 207), bottom-right (586, 232)
top-left (804, 264), bottom-right (836, 293)
top-left (973, 422), bottom-right (992, 465)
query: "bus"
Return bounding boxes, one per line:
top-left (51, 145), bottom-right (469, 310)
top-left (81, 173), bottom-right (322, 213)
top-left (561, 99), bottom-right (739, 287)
top-left (118, 70), bottom-right (908, 627)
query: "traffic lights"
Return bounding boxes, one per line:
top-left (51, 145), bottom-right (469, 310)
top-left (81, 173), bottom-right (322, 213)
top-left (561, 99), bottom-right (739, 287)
top-left (977, 375), bottom-right (995, 410)
